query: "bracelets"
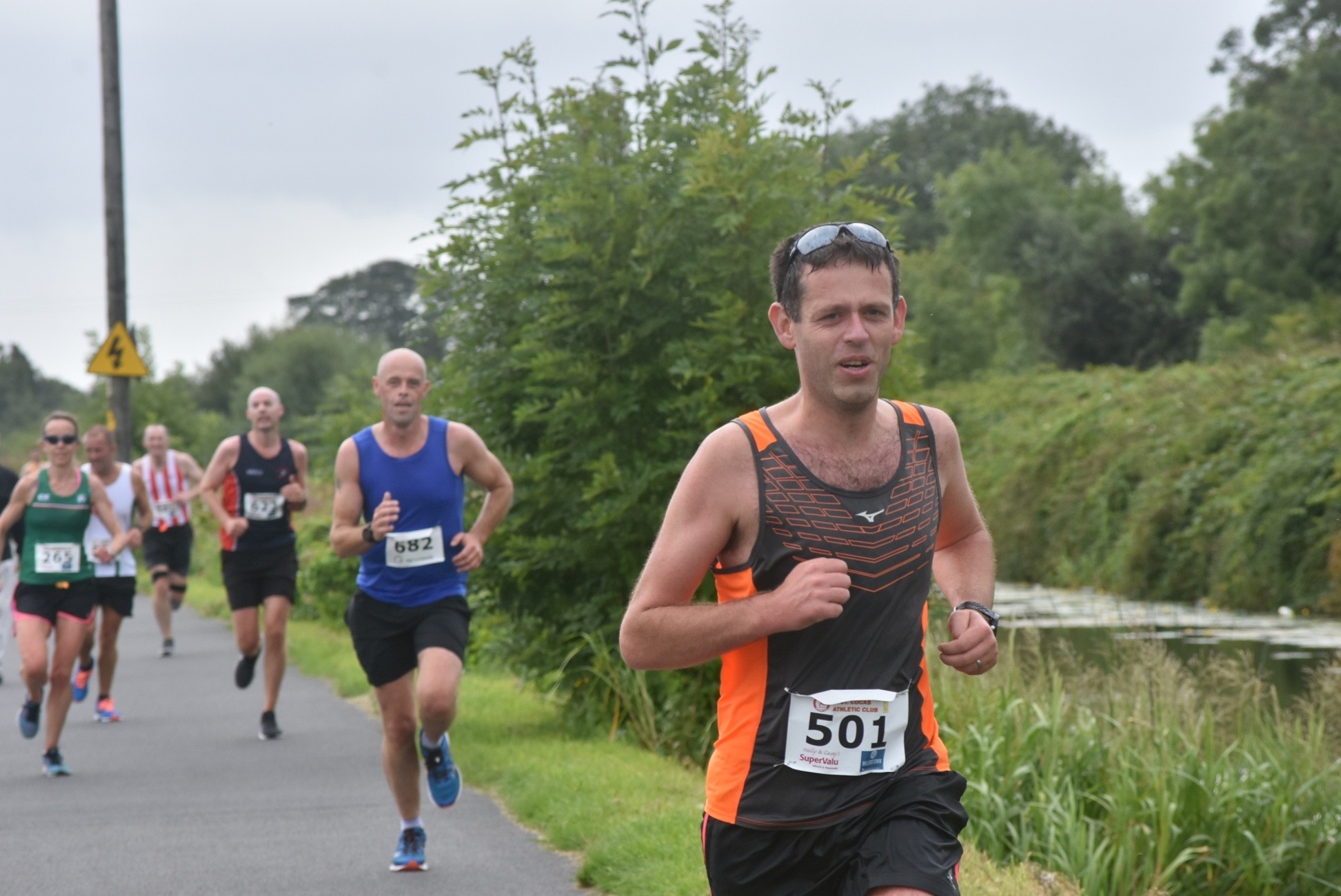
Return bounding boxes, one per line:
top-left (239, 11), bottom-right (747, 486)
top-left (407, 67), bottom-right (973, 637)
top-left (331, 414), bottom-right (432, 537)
top-left (105, 546), bottom-right (118, 559)
top-left (131, 522), bottom-right (147, 534)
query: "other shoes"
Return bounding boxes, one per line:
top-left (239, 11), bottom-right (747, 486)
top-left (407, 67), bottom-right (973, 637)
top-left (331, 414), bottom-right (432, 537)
top-left (163, 640), bottom-right (173, 656)
top-left (170, 597), bottom-right (180, 609)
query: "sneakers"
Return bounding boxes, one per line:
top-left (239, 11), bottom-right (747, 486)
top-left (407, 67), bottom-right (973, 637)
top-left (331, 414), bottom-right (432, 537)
top-left (389, 827), bottom-right (428, 872)
top-left (235, 646), bottom-right (262, 688)
top-left (42, 747), bottom-right (70, 778)
top-left (419, 728), bottom-right (462, 809)
top-left (71, 656), bottom-right (93, 702)
top-left (18, 688), bottom-right (44, 738)
top-left (258, 710), bottom-right (283, 740)
top-left (92, 698), bottom-right (122, 723)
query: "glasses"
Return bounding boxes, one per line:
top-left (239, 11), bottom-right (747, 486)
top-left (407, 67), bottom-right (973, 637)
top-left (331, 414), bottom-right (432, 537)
top-left (45, 435), bottom-right (77, 445)
top-left (776, 222), bottom-right (892, 303)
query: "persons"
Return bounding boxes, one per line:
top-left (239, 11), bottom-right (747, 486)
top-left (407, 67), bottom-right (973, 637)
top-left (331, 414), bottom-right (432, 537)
top-left (132, 423), bottom-right (205, 658)
top-left (331, 348), bottom-right (512, 872)
top-left (618, 221), bottom-right (999, 896)
top-left (0, 411), bottom-right (154, 778)
top-left (199, 386), bottom-right (307, 740)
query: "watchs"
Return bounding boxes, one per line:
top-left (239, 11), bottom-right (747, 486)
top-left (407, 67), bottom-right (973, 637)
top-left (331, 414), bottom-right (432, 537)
top-left (953, 600), bottom-right (999, 636)
top-left (362, 521), bottom-right (381, 543)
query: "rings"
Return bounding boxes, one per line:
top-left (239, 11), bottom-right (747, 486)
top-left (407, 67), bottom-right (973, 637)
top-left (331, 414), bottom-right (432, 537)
top-left (976, 659), bottom-right (984, 671)
